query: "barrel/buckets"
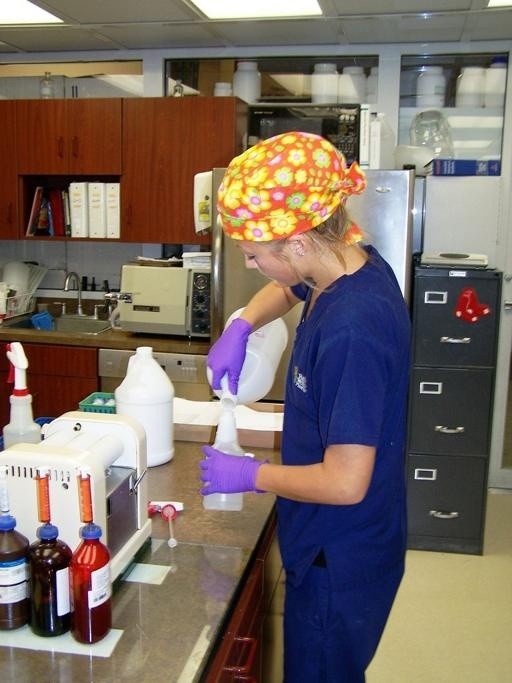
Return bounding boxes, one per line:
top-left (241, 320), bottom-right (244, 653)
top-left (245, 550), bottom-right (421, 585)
top-left (203, 307), bottom-right (289, 409)
top-left (113, 346), bottom-right (176, 469)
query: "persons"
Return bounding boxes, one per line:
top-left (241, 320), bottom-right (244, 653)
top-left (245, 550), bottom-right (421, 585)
top-left (200, 131), bottom-right (414, 682)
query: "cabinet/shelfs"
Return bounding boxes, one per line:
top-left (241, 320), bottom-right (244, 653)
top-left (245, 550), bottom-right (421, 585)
top-left (201, 511), bottom-right (296, 683)
top-left (123, 96), bottom-right (249, 245)
top-left (0, 342), bottom-right (100, 436)
top-left (402, 265), bottom-right (502, 559)
top-left (0, 102), bottom-right (20, 238)
top-left (18, 97), bottom-right (122, 238)
top-left (0, 41), bottom-right (512, 181)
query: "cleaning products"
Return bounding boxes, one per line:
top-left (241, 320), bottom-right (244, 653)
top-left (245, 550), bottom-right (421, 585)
top-left (1, 342), bottom-right (43, 451)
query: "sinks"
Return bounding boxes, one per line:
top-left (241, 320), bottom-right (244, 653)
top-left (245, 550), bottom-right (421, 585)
top-left (3, 314), bottom-right (119, 335)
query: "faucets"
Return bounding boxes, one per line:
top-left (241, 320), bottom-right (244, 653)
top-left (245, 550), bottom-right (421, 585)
top-left (62, 271), bottom-right (86, 316)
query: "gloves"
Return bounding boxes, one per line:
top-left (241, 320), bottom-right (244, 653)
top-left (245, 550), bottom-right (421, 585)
top-left (197, 444), bottom-right (272, 496)
top-left (203, 317), bottom-right (253, 396)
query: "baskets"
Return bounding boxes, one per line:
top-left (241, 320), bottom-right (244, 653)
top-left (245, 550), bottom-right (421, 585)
top-left (78, 391), bottom-right (117, 413)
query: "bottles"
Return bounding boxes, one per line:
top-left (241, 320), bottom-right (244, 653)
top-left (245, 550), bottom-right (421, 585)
top-left (171, 80), bottom-right (184, 98)
top-left (38, 72), bottom-right (56, 99)
top-left (0, 518), bottom-right (114, 645)
top-left (214, 62), bottom-right (507, 111)
